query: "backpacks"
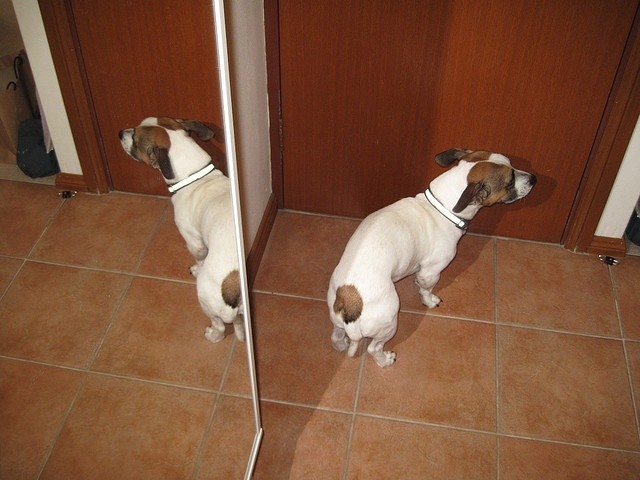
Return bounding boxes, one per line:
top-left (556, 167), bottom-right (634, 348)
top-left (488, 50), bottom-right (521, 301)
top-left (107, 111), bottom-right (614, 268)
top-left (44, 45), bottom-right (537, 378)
top-left (17, 118), bottom-right (59, 178)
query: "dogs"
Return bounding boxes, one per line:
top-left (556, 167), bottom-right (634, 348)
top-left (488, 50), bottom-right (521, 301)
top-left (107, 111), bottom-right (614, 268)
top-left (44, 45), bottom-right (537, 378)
top-left (118, 115), bottom-right (247, 344)
top-left (327, 147), bottom-right (537, 369)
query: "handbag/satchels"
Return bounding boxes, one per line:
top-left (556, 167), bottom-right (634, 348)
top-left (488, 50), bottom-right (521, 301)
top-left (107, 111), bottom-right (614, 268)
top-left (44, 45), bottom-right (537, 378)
top-left (0, 79), bottom-right (35, 165)
top-left (14, 48), bottom-right (41, 116)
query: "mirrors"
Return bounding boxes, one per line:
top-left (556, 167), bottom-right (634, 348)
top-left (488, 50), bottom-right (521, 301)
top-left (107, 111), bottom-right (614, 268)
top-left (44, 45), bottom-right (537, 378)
top-left (0, 0), bottom-right (264, 480)
